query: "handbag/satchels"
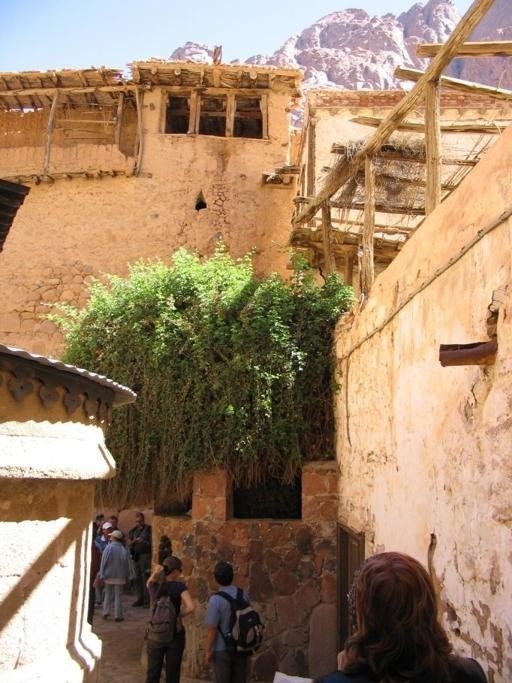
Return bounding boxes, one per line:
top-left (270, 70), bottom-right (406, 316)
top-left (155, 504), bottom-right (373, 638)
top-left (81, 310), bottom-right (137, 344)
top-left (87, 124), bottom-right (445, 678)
top-left (134, 540), bottom-right (150, 553)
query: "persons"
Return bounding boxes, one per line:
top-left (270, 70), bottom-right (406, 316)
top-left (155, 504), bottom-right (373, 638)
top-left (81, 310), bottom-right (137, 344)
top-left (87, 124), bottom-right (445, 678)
top-left (144, 556), bottom-right (195, 683)
top-left (310, 550), bottom-right (488, 683)
top-left (87, 513), bottom-right (152, 625)
top-left (200, 562), bottom-right (253, 683)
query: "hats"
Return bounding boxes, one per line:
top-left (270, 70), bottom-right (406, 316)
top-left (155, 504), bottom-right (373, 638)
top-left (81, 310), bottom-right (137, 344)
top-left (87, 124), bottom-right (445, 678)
top-left (108, 530), bottom-right (123, 540)
top-left (102, 522), bottom-right (112, 529)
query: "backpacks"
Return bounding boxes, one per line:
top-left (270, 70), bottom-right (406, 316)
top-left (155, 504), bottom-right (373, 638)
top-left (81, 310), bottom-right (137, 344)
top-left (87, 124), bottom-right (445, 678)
top-left (215, 589), bottom-right (262, 657)
top-left (145, 583), bottom-right (177, 643)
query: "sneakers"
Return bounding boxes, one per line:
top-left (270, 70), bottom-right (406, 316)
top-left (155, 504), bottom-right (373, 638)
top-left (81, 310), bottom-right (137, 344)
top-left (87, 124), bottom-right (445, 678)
top-left (115, 617), bottom-right (123, 622)
top-left (103, 612), bottom-right (109, 618)
top-left (132, 600), bottom-right (142, 607)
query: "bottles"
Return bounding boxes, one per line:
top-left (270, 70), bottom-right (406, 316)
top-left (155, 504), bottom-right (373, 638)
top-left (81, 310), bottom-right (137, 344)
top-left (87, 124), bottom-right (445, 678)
top-left (225, 633), bottom-right (234, 651)
